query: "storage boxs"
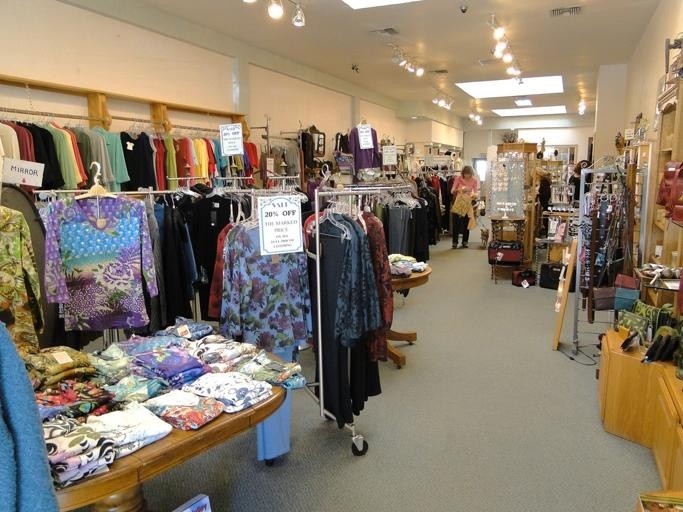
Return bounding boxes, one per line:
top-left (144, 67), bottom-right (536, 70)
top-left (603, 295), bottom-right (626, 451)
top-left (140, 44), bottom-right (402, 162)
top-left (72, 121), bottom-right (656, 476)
top-left (487, 243), bottom-right (524, 265)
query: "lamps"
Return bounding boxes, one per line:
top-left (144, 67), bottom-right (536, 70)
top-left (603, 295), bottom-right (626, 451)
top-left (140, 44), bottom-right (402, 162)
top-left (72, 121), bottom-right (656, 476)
top-left (469, 110), bottom-right (482, 126)
top-left (391, 45), bottom-right (424, 78)
top-left (244, 0), bottom-right (306, 27)
top-left (431, 87), bottom-right (454, 111)
top-left (490, 15), bottom-right (523, 85)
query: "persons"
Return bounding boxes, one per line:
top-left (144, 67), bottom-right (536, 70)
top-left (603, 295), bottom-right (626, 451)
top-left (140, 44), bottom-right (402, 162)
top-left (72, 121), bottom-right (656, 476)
top-left (537, 169), bottom-right (551, 249)
top-left (451, 165), bottom-right (479, 249)
top-left (569, 160), bottom-right (593, 208)
top-left (555, 220), bottom-right (567, 244)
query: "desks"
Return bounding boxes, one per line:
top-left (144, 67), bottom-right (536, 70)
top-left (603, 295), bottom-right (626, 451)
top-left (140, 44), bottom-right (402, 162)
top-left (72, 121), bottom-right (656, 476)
top-left (56, 384), bottom-right (287, 512)
top-left (386, 266), bottom-right (432, 369)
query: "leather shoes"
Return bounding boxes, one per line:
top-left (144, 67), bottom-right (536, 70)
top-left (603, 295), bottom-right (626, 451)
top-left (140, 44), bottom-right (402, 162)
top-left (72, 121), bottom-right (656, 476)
top-left (452, 241), bottom-right (457, 248)
top-left (462, 241), bottom-right (469, 247)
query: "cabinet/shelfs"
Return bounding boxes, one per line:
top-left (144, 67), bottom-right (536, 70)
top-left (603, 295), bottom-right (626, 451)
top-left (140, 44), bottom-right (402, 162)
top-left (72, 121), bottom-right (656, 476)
top-left (598, 330), bottom-right (683, 512)
top-left (631, 79), bottom-right (683, 319)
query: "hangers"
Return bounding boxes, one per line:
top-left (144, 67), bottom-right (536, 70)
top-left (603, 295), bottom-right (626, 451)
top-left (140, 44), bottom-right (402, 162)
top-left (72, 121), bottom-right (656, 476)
top-left (261, 136), bottom-right (300, 157)
top-left (0, 107), bottom-right (103, 136)
top-left (124, 118), bottom-right (220, 153)
top-left (74, 162), bottom-right (118, 200)
top-left (378, 185), bottom-right (428, 210)
top-left (164, 176), bottom-right (308, 229)
top-left (306, 187), bottom-right (383, 241)
top-left (358, 116), bottom-right (368, 125)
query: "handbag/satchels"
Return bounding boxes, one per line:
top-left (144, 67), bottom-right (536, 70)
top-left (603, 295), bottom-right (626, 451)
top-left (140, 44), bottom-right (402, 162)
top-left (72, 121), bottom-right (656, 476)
top-left (594, 242), bottom-right (639, 290)
top-left (593, 268), bottom-right (615, 310)
top-left (656, 161), bottom-right (683, 221)
top-left (450, 189), bottom-right (475, 217)
top-left (616, 287), bottom-right (641, 312)
